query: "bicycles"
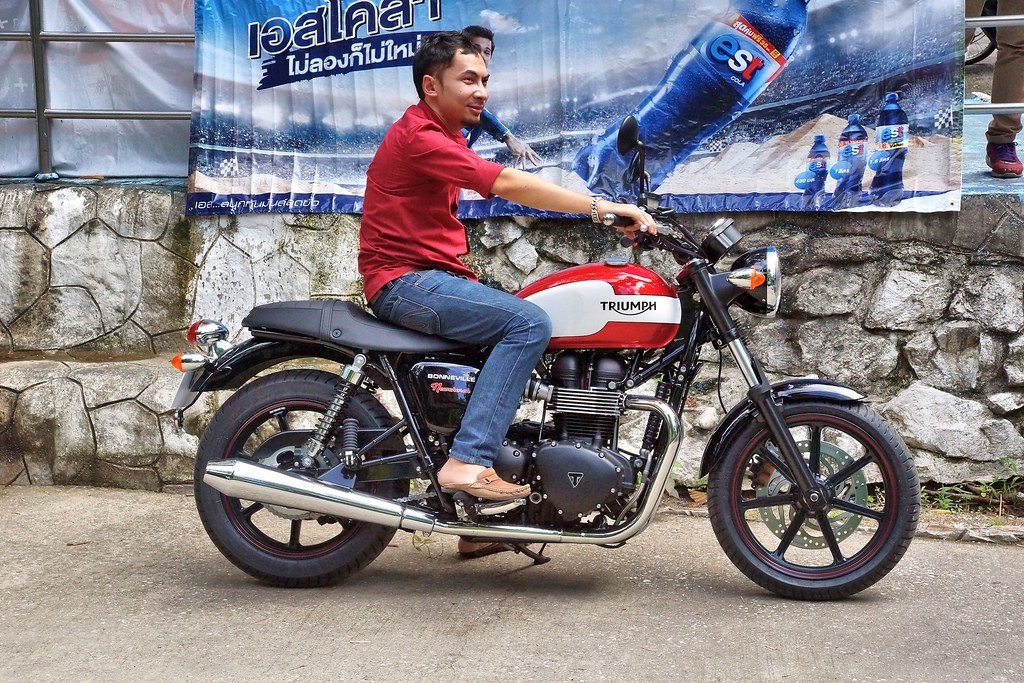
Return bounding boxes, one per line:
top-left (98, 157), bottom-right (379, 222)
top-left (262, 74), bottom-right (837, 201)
top-left (965, 21), bottom-right (996, 65)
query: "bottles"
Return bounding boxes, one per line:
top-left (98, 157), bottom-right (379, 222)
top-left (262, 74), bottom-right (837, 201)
top-left (802, 134), bottom-right (830, 206)
top-left (870, 92), bottom-right (909, 207)
top-left (832, 114), bottom-right (868, 207)
top-left (572, 0), bottom-right (813, 198)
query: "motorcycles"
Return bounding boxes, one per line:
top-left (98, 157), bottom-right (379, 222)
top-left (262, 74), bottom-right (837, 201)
top-left (170, 117), bottom-right (922, 599)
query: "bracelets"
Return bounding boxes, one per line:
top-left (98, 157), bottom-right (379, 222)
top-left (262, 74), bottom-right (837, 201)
top-left (591, 197), bottom-right (602, 222)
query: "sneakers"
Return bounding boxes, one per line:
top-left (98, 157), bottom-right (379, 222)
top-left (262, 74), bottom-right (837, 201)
top-left (986, 139), bottom-right (1022, 179)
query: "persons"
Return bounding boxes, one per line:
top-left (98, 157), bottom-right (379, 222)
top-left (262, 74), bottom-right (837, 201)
top-left (460, 25), bottom-right (541, 171)
top-left (358, 31), bottom-right (657, 500)
top-left (965, 0), bottom-right (1024, 176)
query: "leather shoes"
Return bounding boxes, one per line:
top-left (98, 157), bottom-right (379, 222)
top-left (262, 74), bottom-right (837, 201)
top-left (437, 458), bottom-right (532, 500)
top-left (459, 539), bottom-right (531, 557)
